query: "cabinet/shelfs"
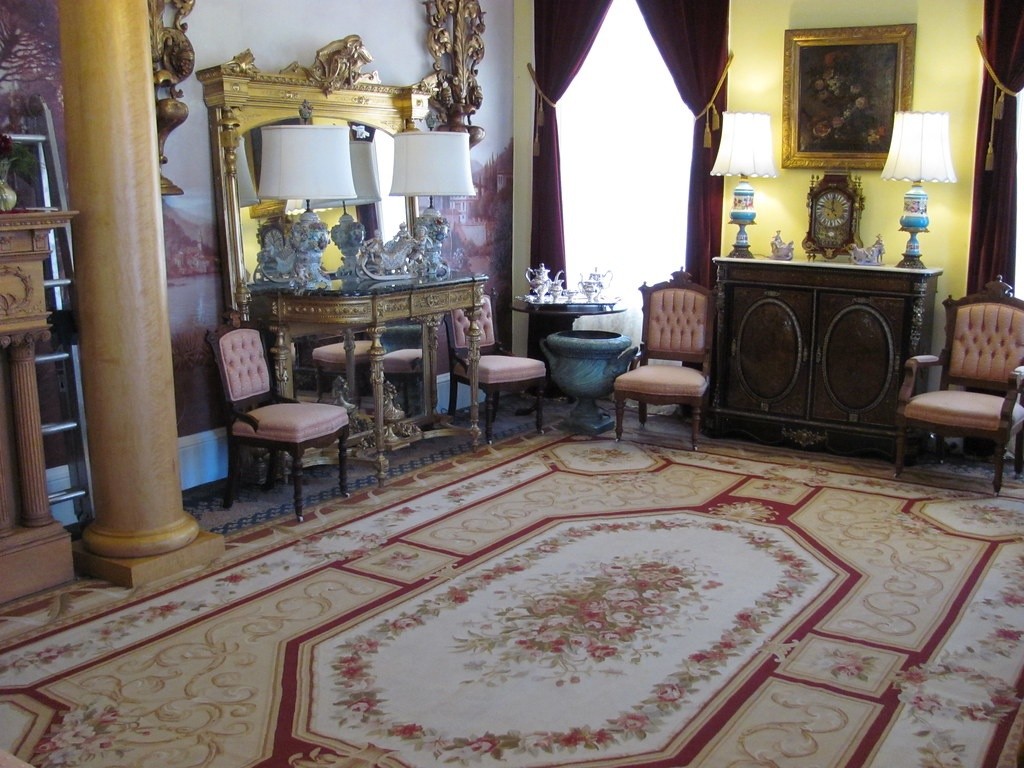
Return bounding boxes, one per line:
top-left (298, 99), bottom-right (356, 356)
top-left (702, 256), bottom-right (944, 468)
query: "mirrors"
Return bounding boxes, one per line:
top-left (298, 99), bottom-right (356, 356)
top-left (196, 34), bottom-right (433, 483)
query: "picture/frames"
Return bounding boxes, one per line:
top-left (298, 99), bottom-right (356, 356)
top-left (782, 23), bottom-right (918, 170)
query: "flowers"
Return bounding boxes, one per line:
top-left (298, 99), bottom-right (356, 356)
top-left (0, 133), bottom-right (38, 186)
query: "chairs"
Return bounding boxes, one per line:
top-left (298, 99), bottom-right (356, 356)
top-left (205, 309), bottom-right (352, 524)
top-left (613, 268), bottom-right (718, 451)
top-left (894, 275), bottom-right (1024, 492)
top-left (312, 339), bottom-right (423, 418)
top-left (445, 288), bottom-right (547, 445)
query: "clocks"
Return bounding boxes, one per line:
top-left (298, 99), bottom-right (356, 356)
top-left (802, 170), bottom-right (864, 262)
top-left (255, 208), bottom-right (294, 255)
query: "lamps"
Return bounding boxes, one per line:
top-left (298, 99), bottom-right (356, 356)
top-left (256, 99), bottom-right (357, 295)
top-left (710, 111), bottom-right (776, 259)
top-left (389, 110), bottom-right (478, 275)
top-left (300, 142), bottom-right (382, 277)
top-left (880, 113), bottom-right (959, 270)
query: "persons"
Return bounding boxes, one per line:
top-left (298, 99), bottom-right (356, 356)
top-left (873, 233), bottom-right (885, 263)
top-left (393, 222), bottom-right (411, 240)
top-left (772, 230), bottom-right (783, 243)
top-left (411, 224), bottom-right (428, 264)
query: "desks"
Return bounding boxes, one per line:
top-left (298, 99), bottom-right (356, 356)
top-left (511, 300), bottom-right (627, 400)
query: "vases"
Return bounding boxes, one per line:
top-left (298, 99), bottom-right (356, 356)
top-left (0, 161), bottom-right (17, 212)
